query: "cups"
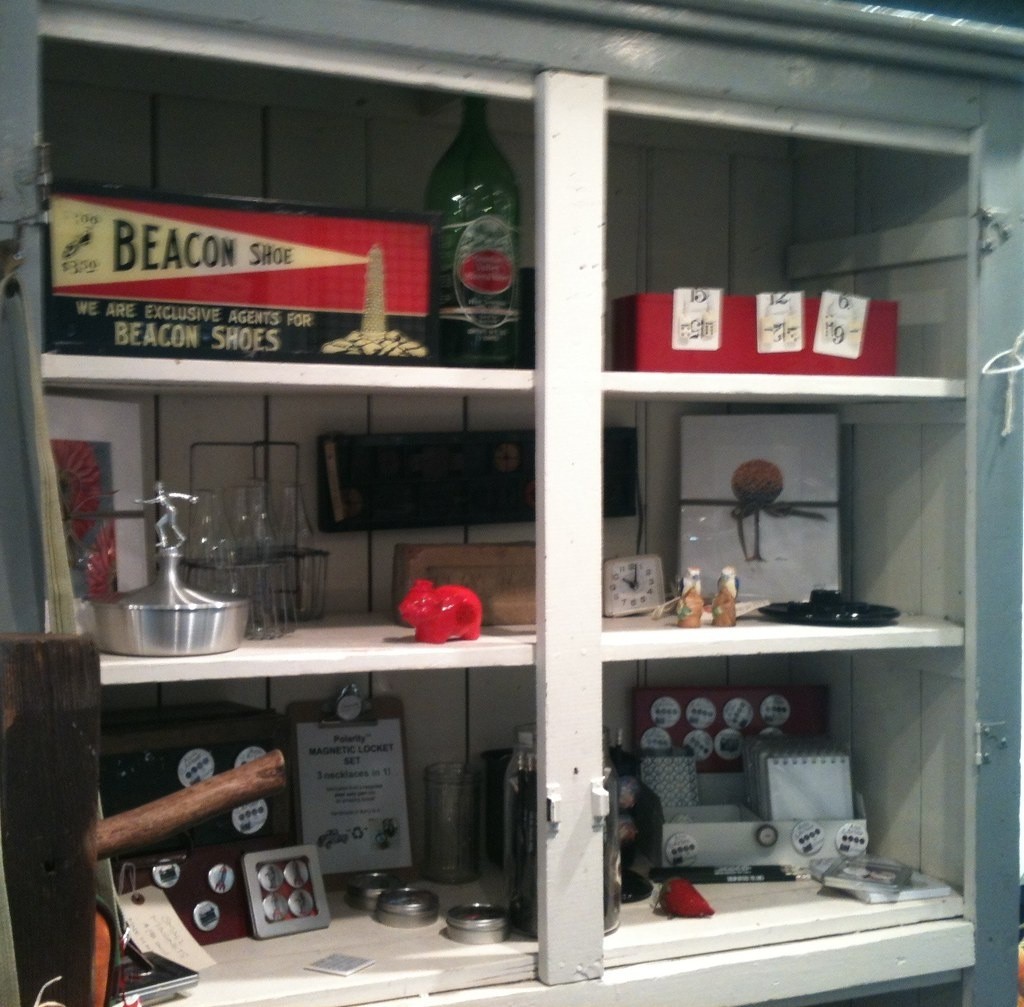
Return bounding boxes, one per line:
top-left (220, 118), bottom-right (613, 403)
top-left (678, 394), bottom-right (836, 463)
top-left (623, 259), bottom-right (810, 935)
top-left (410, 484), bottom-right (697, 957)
top-left (422, 761), bottom-right (484, 885)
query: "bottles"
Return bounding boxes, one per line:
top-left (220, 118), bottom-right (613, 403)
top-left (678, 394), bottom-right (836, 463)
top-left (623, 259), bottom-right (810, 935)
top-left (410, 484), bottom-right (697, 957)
top-left (425, 97), bottom-right (523, 369)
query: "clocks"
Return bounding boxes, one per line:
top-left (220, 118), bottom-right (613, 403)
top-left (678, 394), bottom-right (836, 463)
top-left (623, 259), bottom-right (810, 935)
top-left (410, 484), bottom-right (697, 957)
top-left (604, 555), bottom-right (666, 616)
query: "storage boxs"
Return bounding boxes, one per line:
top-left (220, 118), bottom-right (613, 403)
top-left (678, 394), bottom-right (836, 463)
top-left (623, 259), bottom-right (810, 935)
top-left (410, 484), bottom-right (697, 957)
top-left (655, 772), bottom-right (872, 870)
top-left (99, 703), bottom-right (289, 858)
top-left (612, 293), bottom-right (898, 375)
top-left (0, 0), bottom-right (1024, 1007)
top-left (40, 182), bottom-right (440, 367)
top-left (42, 386), bottom-right (156, 596)
top-left (392, 543), bottom-right (537, 627)
